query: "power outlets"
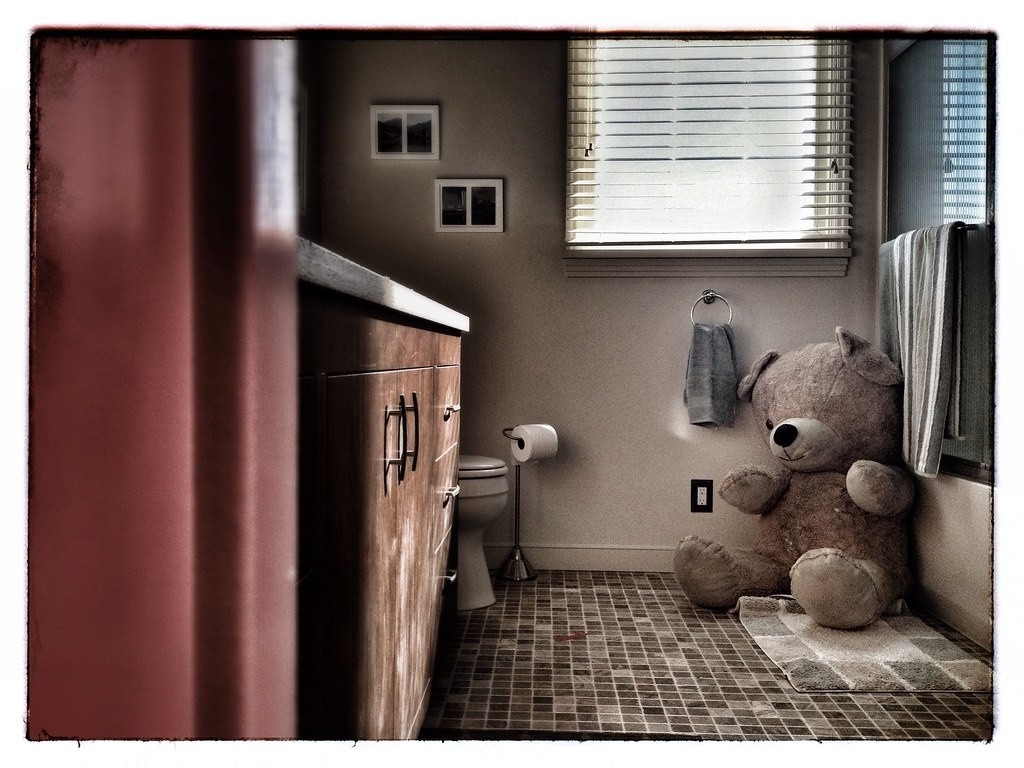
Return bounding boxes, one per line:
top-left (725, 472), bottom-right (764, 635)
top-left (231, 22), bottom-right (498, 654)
top-left (691, 478), bottom-right (714, 513)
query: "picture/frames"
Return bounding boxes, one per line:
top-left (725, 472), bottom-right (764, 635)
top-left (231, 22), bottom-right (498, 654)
top-left (434, 179), bottom-right (504, 233)
top-left (369, 104), bottom-right (440, 161)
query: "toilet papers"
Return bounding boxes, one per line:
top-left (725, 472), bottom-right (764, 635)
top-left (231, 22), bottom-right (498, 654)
top-left (509, 423), bottom-right (559, 466)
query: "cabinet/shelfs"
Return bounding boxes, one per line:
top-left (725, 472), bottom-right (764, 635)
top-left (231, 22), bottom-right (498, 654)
top-left (252, 285), bottom-right (462, 740)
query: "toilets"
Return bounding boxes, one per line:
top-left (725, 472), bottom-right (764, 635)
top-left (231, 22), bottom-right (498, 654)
top-left (454, 453), bottom-right (511, 611)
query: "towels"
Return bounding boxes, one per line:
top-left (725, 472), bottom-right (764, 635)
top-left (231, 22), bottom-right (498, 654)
top-left (875, 221), bottom-right (969, 479)
top-left (681, 320), bottom-right (741, 431)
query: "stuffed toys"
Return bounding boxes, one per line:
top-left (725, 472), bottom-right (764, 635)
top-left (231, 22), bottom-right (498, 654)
top-left (673, 325), bottom-right (914, 630)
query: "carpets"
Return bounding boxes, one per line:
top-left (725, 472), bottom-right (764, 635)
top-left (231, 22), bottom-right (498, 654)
top-left (727, 594), bottom-right (993, 694)
